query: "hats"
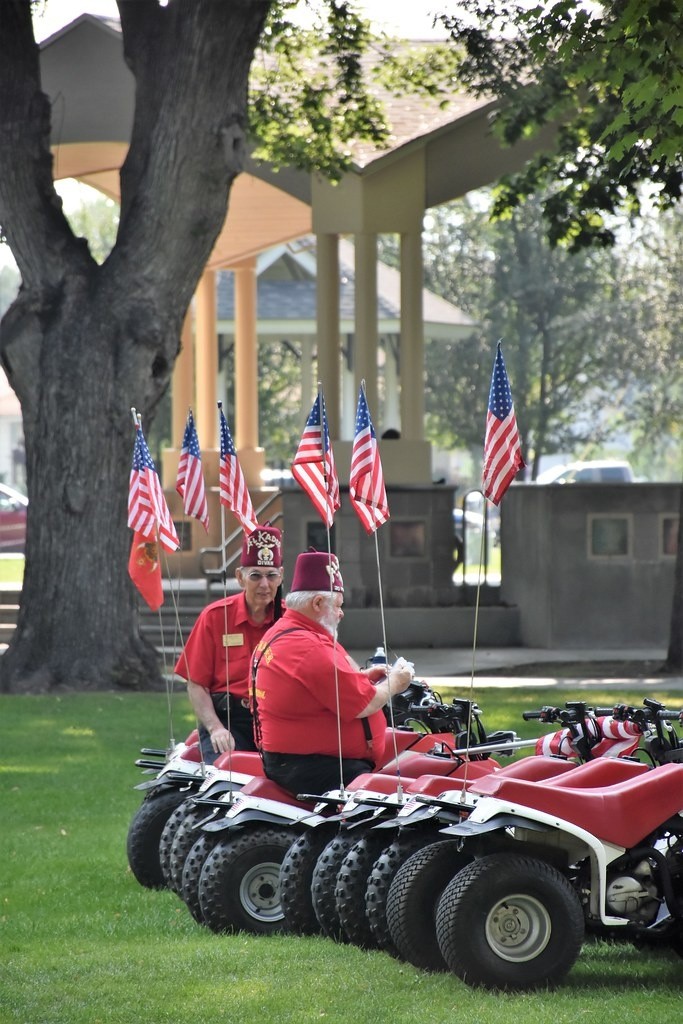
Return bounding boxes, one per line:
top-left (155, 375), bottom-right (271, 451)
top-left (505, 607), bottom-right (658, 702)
top-left (290, 546), bottom-right (344, 593)
top-left (240, 520), bottom-right (282, 567)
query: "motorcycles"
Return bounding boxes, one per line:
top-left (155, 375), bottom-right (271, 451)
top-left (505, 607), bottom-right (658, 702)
top-left (125, 678), bottom-right (604, 965)
top-left (367, 697), bottom-right (683, 996)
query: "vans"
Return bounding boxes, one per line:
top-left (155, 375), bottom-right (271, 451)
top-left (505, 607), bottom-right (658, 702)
top-left (537, 462), bottom-right (632, 485)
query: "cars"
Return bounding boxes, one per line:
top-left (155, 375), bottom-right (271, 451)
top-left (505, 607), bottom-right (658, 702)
top-left (0, 481), bottom-right (28, 553)
top-left (449, 506), bottom-right (497, 576)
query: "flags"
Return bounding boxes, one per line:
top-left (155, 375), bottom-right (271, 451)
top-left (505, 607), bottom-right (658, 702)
top-left (218, 412), bottom-right (257, 538)
top-left (349, 384), bottom-right (389, 534)
top-left (126, 425), bottom-right (181, 612)
top-left (479, 342), bottom-right (525, 508)
top-left (175, 409), bottom-right (211, 534)
top-left (290, 390), bottom-right (340, 529)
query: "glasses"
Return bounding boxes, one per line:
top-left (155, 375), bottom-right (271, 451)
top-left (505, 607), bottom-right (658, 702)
top-left (242, 571), bottom-right (281, 582)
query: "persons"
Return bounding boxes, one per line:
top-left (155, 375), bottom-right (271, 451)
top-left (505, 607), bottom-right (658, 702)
top-left (173, 521), bottom-right (286, 766)
top-left (248, 543), bottom-right (414, 801)
